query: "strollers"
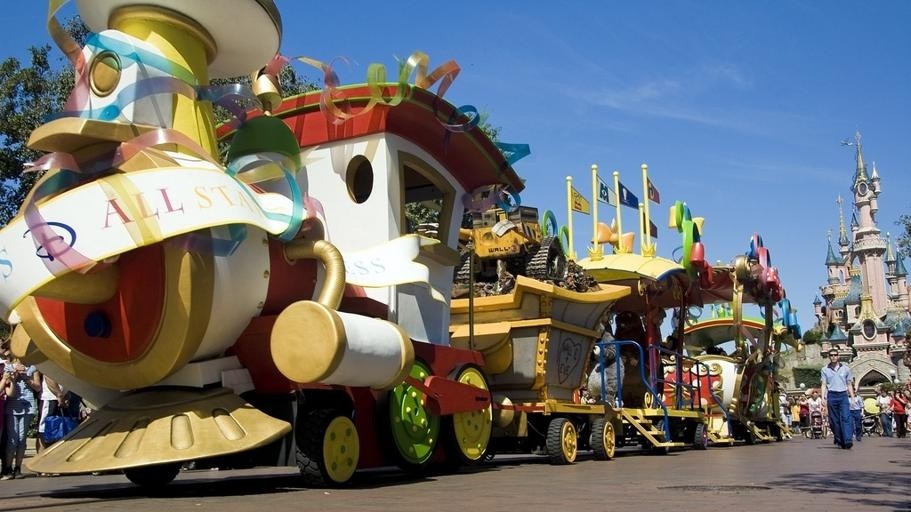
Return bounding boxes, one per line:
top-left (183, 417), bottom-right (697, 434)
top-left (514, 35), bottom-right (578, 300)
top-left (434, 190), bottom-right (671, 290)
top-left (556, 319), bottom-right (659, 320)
top-left (810, 410), bottom-right (824, 440)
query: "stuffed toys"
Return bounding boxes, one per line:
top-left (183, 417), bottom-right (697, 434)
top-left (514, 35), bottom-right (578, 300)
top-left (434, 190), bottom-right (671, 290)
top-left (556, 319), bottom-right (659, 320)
top-left (581, 324), bottom-right (627, 409)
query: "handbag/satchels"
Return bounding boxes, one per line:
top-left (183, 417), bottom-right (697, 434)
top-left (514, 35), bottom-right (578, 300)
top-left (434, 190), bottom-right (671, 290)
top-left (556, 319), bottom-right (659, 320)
top-left (41, 408), bottom-right (82, 444)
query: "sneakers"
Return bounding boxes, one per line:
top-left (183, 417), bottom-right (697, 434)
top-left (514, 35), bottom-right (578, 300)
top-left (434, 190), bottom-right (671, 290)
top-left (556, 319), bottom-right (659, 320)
top-left (1, 469), bottom-right (25, 481)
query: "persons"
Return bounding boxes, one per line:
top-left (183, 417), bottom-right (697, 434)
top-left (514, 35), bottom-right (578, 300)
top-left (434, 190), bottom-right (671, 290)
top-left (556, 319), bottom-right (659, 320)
top-left (820, 349), bottom-right (858, 452)
top-left (780, 385), bottom-right (911, 438)
top-left (0, 341), bottom-right (95, 481)
